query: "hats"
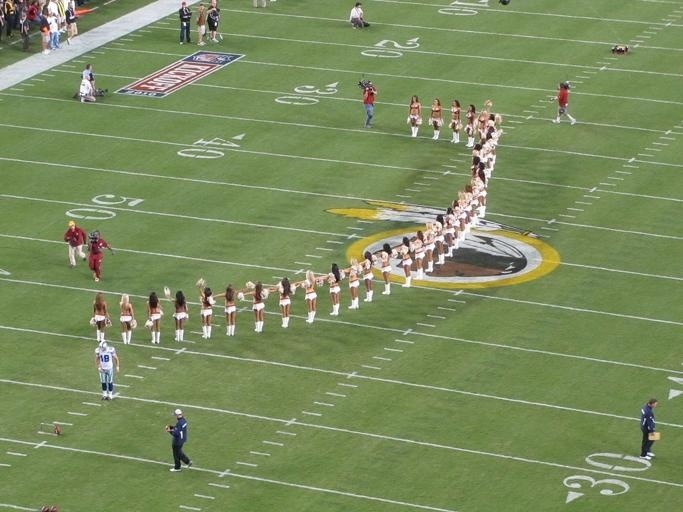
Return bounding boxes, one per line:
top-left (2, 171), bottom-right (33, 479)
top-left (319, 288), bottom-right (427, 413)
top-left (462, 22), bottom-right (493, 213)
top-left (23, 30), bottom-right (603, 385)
top-left (172, 409), bottom-right (182, 415)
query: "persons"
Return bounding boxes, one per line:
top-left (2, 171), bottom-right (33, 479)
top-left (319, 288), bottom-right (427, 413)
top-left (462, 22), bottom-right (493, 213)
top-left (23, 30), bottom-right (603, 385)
top-left (550, 82), bottom-right (576, 126)
top-left (196, 4), bottom-right (207, 47)
top-left (206, 0), bottom-right (220, 43)
top-left (349, 2), bottom-right (370, 30)
top-left (640, 398), bottom-right (659, 462)
top-left (179, 1), bottom-right (195, 45)
top-left (0, 0), bottom-right (81, 56)
top-left (164, 408), bottom-right (195, 475)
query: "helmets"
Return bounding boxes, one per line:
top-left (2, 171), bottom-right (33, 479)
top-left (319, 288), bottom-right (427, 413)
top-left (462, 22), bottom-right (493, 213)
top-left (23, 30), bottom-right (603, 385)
top-left (98, 340), bottom-right (107, 351)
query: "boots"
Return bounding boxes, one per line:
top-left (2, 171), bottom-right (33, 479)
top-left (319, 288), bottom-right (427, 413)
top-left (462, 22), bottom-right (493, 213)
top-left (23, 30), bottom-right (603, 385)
top-left (226, 325), bottom-right (235, 335)
top-left (97, 330), bottom-right (104, 342)
top-left (281, 317), bottom-right (290, 327)
top-left (330, 304), bottom-right (339, 316)
top-left (363, 290), bottom-right (373, 301)
top-left (202, 326), bottom-right (211, 339)
top-left (432, 130), bottom-right (439, 140)
top-left (465, 136), bottom-right (475, 148)
top-left (382, 282), bottom-right (391, 295)
top-left (152, 331), bottom-right (160, 344)
top-left (255, 321), bottom-right (263, 333)
top-left (174, 329), bottom-right (184, 342)
top-left (411, 127), bottom-right (418, 137)
top-left (403, 155), bottom-right (497, 287)
top-left (306, 311), bottom-right (315, 324)
top-left (122, 331), bottom-right (131, 345)
top-left (348, 297), bottom-right (359, 309)
top-left (451, 132), bottom-right (459, 143)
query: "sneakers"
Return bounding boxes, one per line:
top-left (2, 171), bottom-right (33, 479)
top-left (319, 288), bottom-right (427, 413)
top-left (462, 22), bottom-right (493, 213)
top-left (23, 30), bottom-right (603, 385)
top-left (640, 452), bottom-right (656, 460)
top-left (169, 461), bottom-right (192, 472)
top-left (102, 393), bottom-right (112, 400)
